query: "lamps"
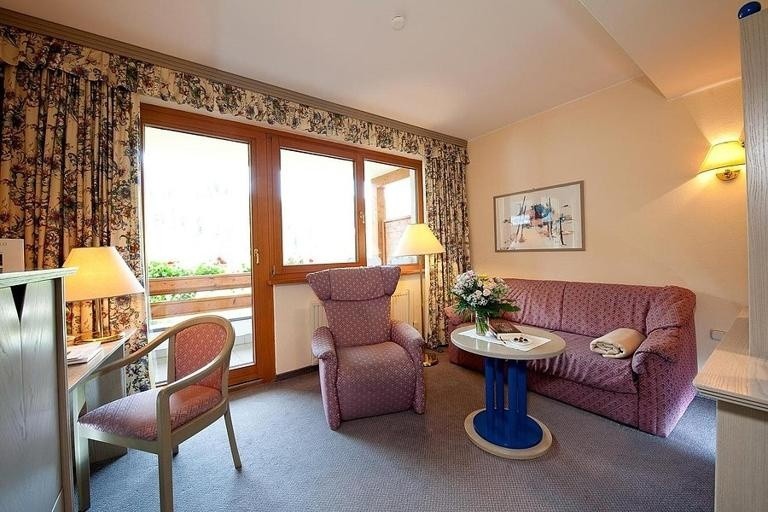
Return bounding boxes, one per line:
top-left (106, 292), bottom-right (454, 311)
top-left (696, 141), bottom-right (747, 182)
top-left (63, 246), bottom-right (145, 342)
top-left (391, 225), bottom-right (447, 367)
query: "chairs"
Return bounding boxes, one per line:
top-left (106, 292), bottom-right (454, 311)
top-left (74, 313), bottom-right (240, 511)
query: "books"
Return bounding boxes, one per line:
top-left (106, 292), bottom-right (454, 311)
top-left (489, 320), bottom-right (522, 340)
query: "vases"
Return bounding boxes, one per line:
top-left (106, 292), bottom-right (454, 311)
top-left (475, 312), bottom-right (491, 335)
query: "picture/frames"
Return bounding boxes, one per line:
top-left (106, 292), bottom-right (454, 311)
top-left (492, 180), bottom-right (586, 253)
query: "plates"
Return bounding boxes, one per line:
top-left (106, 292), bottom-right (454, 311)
top-left (510, 336), bottom-right (533, 345)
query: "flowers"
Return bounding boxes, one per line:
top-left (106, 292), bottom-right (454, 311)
top-left (448, 270), bottom-right (521, 329)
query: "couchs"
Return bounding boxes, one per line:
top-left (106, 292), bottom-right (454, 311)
top-left (304, 266), bottom-right (428, 431)
top-left (442, 277), bottom-right (698, 439)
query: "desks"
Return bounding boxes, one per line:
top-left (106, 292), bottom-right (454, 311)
top-left (451, 324), bottom-right (566, 462)
top-left (63, 327), bottom-right (138, 510)
